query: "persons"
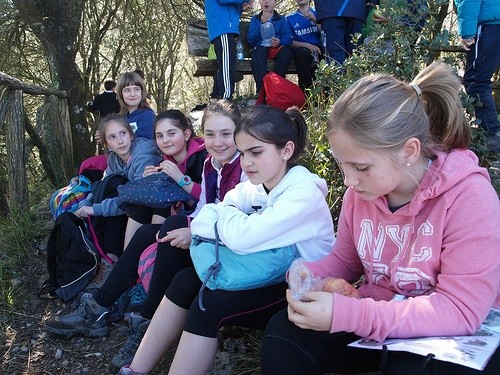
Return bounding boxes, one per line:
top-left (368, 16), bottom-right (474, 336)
top-left (119, 107), bottom-right (335, 372)
top-left (261, 62), bottom-right (500, 374)
top-left (38, 70), bottom-right (206, 300)
top-left (204, 1), bottom-right (500, 136)
top-left (46, 99), bottom-right (242, 362)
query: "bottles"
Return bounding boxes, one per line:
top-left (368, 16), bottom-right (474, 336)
top-left (236, 39), bottom-right (245, 61)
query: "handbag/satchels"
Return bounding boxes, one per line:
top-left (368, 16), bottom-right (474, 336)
top-left (117, 169), bottom-right (198, 224)
top-left (49, 175), bottom-right (94, 221)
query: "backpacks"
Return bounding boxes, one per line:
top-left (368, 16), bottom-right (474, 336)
top-left (257, 71), bottom-right (305, 111)
top-left (47, 211), bottom-right (102, 303)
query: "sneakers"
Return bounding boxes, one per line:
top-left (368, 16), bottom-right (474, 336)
top-left (43, 294), bottom-right (120, 338)
top-left (473, 133), bottom-right (500, 154)
top-left (37, 282), bottom-right (61, 301)
top-left (107, 312), bottom-right (151, 372)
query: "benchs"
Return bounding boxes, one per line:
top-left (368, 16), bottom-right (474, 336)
top-left (186, 19), bottom-right (297, 97)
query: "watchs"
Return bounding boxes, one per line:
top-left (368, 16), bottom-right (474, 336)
top-left (178, 175), bottom-right (192, 188)
